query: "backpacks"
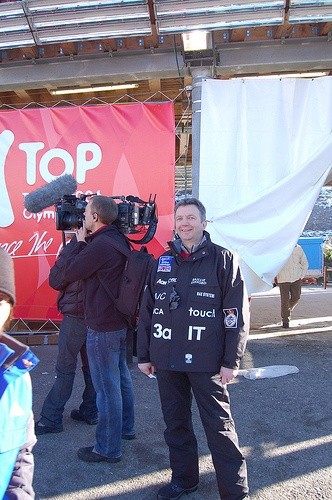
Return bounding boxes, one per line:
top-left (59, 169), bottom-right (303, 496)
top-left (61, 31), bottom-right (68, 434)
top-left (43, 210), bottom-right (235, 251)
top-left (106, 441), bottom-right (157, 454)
top-left (87, 236), bottom-right (157, 317)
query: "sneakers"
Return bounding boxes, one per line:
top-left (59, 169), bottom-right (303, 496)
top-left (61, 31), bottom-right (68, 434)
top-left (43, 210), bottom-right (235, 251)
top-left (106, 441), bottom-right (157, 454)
top-left (34, 421), bottom-right (63, 434)
top-left (157, 481), bottom-right (200, 500)
top-left (78, 447), bottom-right (121, 463)
top-left (121, 432), bottom-right (137, 439)
top-left (71, 409), bottom-right (101, 424)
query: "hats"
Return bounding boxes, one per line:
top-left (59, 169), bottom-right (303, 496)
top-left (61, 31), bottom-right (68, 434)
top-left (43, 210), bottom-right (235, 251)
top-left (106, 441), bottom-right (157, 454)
top-left (0, 246), bottom-right (15, 306)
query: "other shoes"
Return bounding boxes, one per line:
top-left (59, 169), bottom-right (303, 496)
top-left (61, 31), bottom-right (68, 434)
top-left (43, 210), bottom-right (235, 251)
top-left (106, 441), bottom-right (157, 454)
top-left (283, 322), bottom-right (289, 328)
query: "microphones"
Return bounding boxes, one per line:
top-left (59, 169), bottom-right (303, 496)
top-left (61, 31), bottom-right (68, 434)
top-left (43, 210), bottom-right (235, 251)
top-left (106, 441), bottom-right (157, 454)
top-left (24, 174), bottom-right (78, 214)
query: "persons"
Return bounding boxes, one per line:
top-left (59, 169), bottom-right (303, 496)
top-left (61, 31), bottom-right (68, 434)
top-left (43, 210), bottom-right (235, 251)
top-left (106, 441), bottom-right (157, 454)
top-left (64, 195), bottom-right (136, 463)
top-left (33, 233), bottom-right (98, 435)
top-left (275, 243), bottom-right (309, 328)
top-left (137, 200), bottom-right (251, 500)
top-left (0, 245), bottom-right (40, 500)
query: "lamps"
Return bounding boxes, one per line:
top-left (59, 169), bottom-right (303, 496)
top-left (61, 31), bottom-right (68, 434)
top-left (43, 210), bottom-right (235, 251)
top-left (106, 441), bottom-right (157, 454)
top-left (50, 84), bottom-right (139, 96)
top-left (179, 30), bottom-right (220, 66)
